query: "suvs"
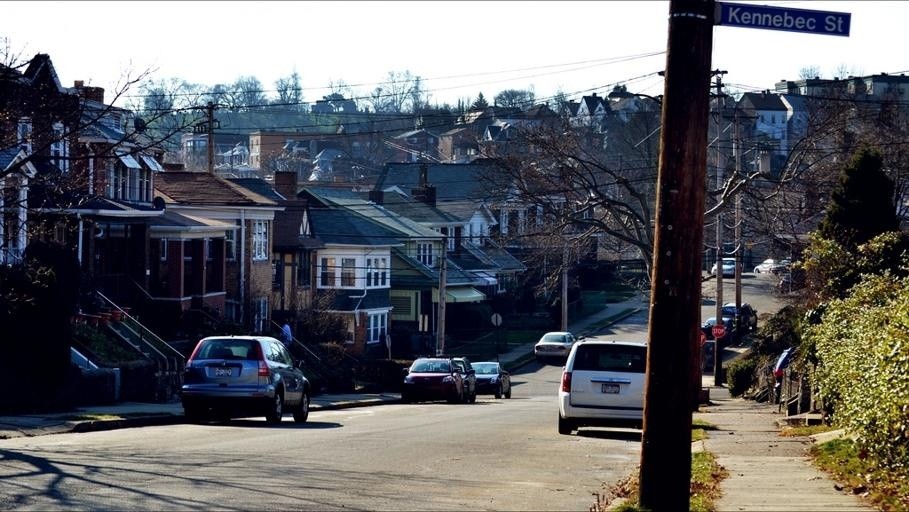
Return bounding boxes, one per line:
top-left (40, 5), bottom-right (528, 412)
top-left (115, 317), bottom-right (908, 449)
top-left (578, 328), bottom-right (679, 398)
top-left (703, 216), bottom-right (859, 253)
top-left (554, 335), bottom-right (649, 438)
top-left (701, 298), bottom-right (758, 376)
top-left (180, 333), bottom-right (311, 430)
top-left (772, 344), bottom-right (818, 416)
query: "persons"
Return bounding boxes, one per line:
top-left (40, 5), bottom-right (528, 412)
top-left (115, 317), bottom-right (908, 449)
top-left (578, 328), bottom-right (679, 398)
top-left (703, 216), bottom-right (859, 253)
top-left (279, 319), bottom-right (292, 342)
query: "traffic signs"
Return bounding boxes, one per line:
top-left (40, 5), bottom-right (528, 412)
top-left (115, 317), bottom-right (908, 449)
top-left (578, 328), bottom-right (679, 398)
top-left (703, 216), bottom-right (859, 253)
top-left (714, 0), bottom-right (852, 39)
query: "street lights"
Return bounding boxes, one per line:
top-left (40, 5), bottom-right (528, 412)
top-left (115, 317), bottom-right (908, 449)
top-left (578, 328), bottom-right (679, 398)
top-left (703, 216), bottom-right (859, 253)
top-left (602, 88), bottom-right (662, 112)
top-left (561, 232), bottom-right (606, 333)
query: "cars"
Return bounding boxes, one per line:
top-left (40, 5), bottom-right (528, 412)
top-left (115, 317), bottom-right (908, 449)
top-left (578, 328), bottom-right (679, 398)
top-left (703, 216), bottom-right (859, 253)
top-left (399, 355), bottom-right (515, 402)
top-left (710, 255), bottom-right (804, 292)
top-left (533, 328), bottom-right (581, 362)
